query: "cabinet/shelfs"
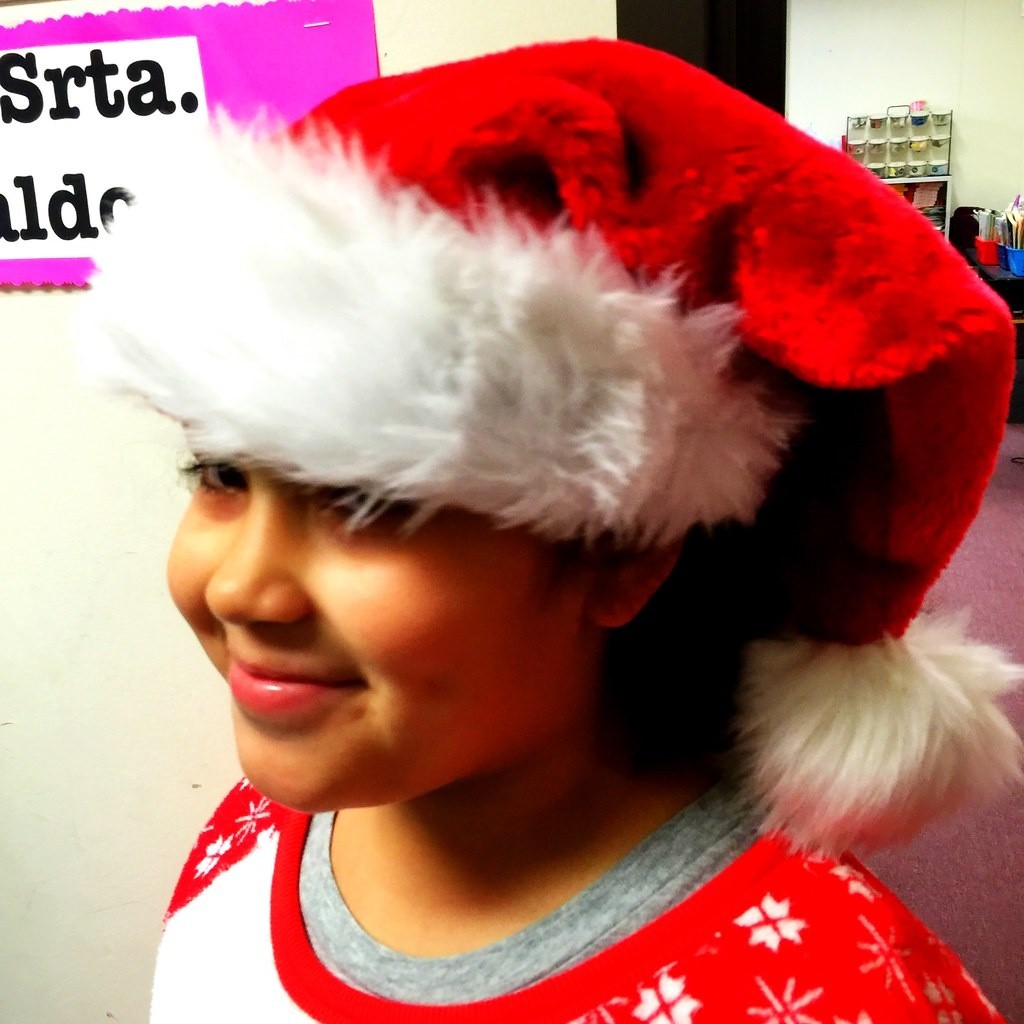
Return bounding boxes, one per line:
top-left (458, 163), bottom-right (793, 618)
top-left (967, 247), bottom-right (1024, 423)
top-left (879, 175), bottom-right (951, 239)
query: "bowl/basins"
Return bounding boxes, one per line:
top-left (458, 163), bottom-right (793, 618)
top-left (846, 101), bottom-right (949, 176)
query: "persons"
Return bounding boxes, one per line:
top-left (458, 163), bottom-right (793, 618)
top-left (85, 42), bottom-right (1023, 1024)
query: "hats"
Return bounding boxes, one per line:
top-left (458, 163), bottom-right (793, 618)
top-left (86, 43), bottom-right (1024, 857)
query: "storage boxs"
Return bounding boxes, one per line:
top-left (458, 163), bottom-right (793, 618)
top-left (996, 242), bottom-right (1010, 270)
top-left (1005, 246), bottom-right (1023, 276)
top-left (974, 236), bottom-right (998, 265)
